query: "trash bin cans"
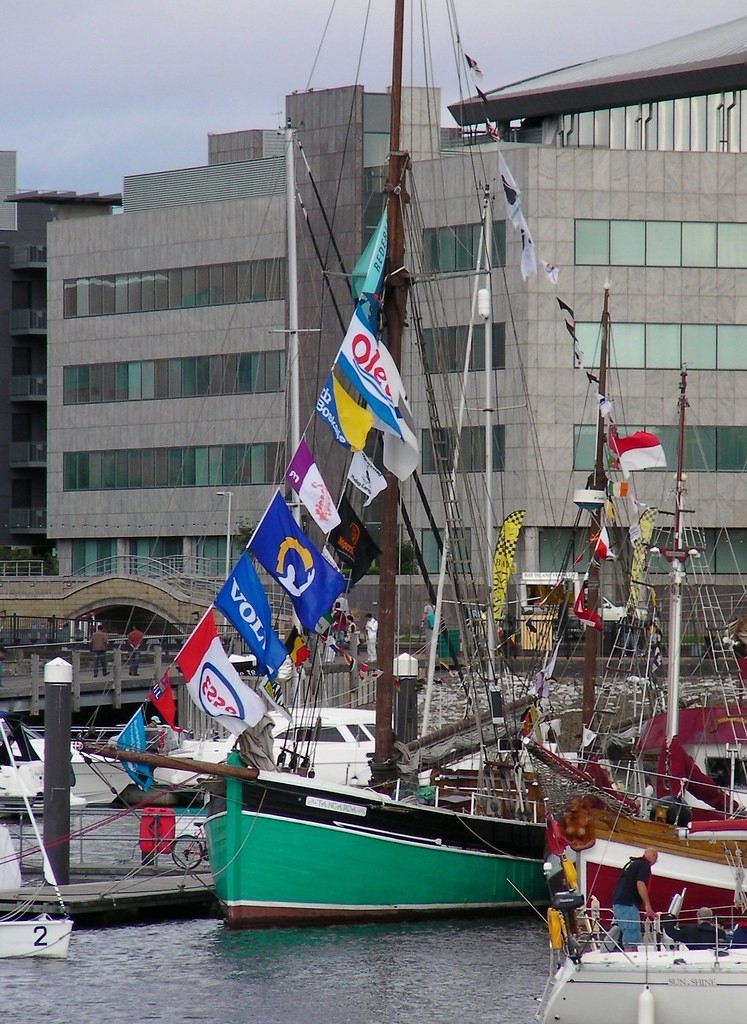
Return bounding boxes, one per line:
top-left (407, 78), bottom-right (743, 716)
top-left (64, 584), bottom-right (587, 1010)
top-left (139, 808), bottom-right (175, 865)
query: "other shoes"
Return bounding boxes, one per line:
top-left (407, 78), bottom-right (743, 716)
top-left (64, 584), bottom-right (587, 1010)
top-left (94, 675), bottom-right (98, 677)
top-left (103, 672), bottom-right (110, 676)
top-left (129, 673), bottom-right (138, 676)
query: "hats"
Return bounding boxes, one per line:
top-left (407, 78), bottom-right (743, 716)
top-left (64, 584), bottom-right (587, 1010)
top-left (151, 716), bottom-right (161, 723)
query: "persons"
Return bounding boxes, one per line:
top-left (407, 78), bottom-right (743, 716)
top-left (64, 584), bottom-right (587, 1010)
top-left (420, 598), bottom-right (436, 629)
top-left (365, 613), bottom-right (379, 663)
top-left (610, 846), bottom-right (660, 952)
top-left (632, 774), bottom-right (654, 804)
top-left (664, 906), bottom-right (727, 950)
top-left (0, 735), bottom-right (16, 764)
top-left (143, 716), bottom-right (160, 783)
top-left (90, 624), bottom-right (111, 678)
top-left (127, 624), bottom-right (144, 676)
top-left (330, 590), bottom-right (362, 656)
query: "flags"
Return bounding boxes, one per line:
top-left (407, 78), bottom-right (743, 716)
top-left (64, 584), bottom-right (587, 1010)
top-left (326, 494), bottom-right (381, 588)
top-left (116, 708), bottom-right (152, 793)
top-left (606, 429), bottom-right (668, 478)
top-left (212, 550), bottom-right (291, 687)
top-left (245, 487), bottom-right (347, 636)
top-left (280, 434), bottom-right (343, 535)
top-left (146, 666), bottom-right (183, 733)
top-left (311, 202), bottom-right (422, 508)
top-left (175, 606), bottom-right (266, 737)
top-left (457, 50), bottom-right (671, 630)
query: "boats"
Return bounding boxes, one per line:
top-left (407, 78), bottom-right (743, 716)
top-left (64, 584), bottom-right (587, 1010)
top-left (8, 707), bottom-right (446, 805)
top-left (0, 717), bottom-right (74, 958)
top-left (0, 710), bottom-right (89, 818)
top-left (533, 889), bottom-right (747, 1023)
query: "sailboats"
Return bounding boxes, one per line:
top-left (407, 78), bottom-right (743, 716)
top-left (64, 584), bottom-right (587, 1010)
top-left (203, 2), bottom-right (569, 930)
top-left (520, 363), bottom-right (747, 933)
